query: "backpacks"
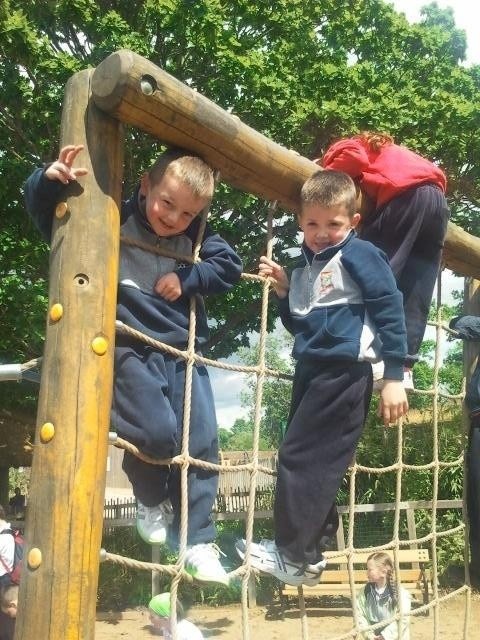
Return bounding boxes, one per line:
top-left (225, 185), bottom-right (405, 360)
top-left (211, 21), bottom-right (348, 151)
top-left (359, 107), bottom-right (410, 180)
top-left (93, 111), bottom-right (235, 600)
top-left (0, 526), bottom-right (24, 585)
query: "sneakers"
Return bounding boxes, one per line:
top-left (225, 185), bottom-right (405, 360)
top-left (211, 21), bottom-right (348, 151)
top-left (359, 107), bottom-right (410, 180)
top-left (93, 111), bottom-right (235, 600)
top-left (186, 544), bottom-right (230, 588)
top-left (446, 564), bottom-right (480, 592)
top-left (234, 538), bottom-right (327, 586)
top-left (371, 359), bottom-right (415, 391)
top-left (135, 499), bottom-right (168, 545)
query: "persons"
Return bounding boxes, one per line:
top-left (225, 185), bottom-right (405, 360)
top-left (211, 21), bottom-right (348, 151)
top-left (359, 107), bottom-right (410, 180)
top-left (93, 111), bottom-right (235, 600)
top-left (445, 313), bottom-right (480, 594)
top-left (1, 504), bottom-right (23, 640)
top-left (236, 164), bottom-right (411, 587)
top-left (1, 582), bottom-right (21, 619)
top-left (354, 549), bottom-right (412, 640)
top-left (23, 143), bottom-right (243, 590)
top-left (147, 591), bottom-right (205, 640)
top-left (8, 486), bottom-right (26, 517)
top-left (311, 129), bottom-right (451, 394)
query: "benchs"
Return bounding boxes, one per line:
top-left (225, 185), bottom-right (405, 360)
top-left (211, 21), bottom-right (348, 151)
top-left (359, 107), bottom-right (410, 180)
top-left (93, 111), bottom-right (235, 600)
top-left (279, 549), bottom-right (432, 617)
